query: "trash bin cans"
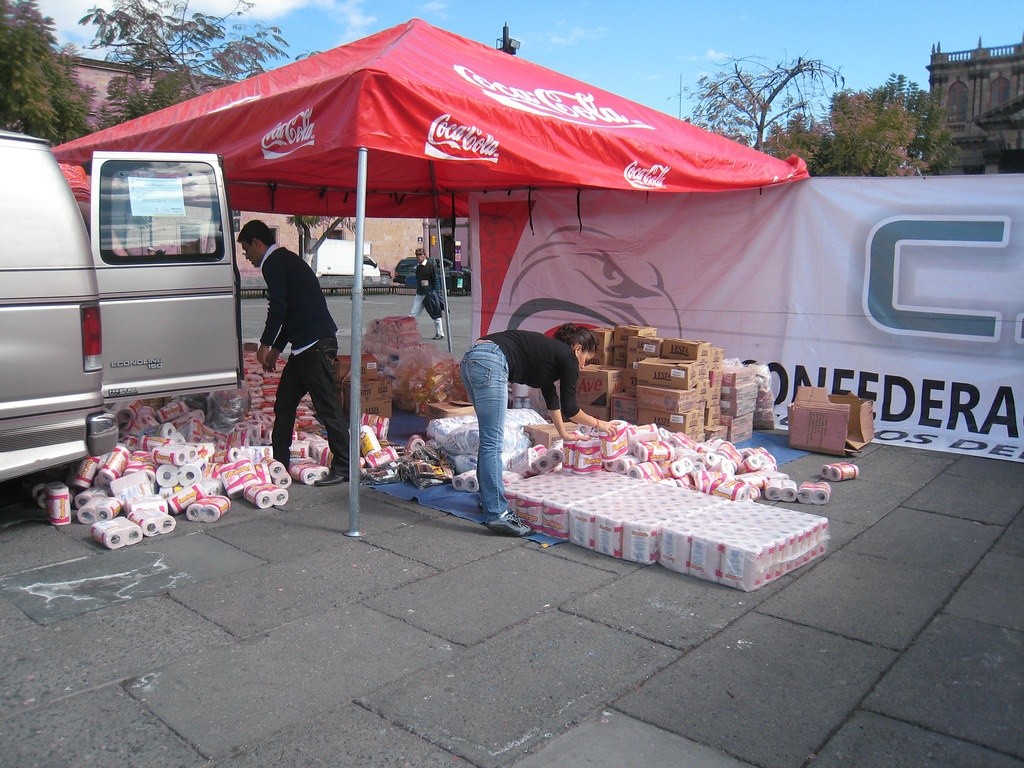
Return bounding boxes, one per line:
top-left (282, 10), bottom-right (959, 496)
top-left (451, 271), bottom-right (463, 292)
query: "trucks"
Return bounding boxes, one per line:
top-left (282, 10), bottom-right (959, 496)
top-left (311, 238), bottom-right (381, 282)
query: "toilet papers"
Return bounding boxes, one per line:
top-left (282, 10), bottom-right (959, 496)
top-left (29, 315), bottom-right (861, 595)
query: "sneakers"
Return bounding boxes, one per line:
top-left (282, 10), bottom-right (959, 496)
top-left (487, 508), bottom-right (535, 536)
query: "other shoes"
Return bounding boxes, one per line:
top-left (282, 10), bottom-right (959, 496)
top-left (433, 335), bottom-right (442, 340)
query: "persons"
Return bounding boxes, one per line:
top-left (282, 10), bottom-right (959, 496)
top-left (461, 323), bottom-right (619, 535)
top-left (237, 220), bottom-right (349, 486)
top-left (409, 248), bottom-right (444, 339)
top-left (58, 163), bottom-right (129, 256)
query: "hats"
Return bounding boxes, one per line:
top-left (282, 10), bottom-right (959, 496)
top-left (416, 248), bottom-right (424, 255)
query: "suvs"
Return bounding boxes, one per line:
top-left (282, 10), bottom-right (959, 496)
top-left (393, 257), bottom-right (471, 284)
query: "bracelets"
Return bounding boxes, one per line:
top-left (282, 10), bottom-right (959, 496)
top-left (595, 419), bottom-right (599, 429)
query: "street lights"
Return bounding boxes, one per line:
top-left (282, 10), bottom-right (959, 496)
top-left (299, 230), bottom-right (303, 260)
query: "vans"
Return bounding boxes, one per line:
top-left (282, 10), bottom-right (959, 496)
top-left (0, 128), bottom-right (241, 490)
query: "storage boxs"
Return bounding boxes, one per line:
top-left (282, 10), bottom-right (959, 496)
top-left (524, 419), bottom-right (578, 450)
top-left (424, 400), bottom-right (474, 428)
top-left (787, 393), bottom-right (875, 450)
top-left (334, 350), bottom-right (394, 418)
top-left (569, 324), bottom-right (758, 445)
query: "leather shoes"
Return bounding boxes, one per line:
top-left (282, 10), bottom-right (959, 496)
top-left (315, 473), bottom-right (348, 485)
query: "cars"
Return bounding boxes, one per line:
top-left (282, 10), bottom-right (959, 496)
top-left (381, 270), bottom-right (392, 281)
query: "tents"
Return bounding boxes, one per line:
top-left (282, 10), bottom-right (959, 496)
top-left (48, 19), bottom-right (810, 537)
